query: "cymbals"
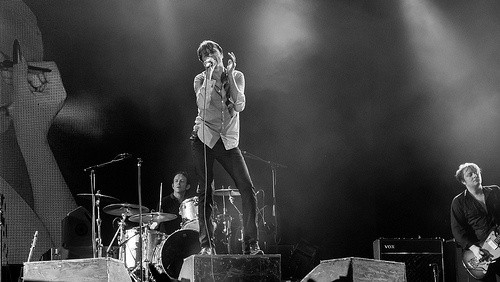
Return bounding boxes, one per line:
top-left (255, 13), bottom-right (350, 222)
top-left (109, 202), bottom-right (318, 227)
top-left (213, 189), bottom-right (242, 197)
top-left (77, 194), bottom-right (120, 202)
top-left (128, 212), bottom-right (177, 222)
top-left (103, 204), bottom-right (149, 216)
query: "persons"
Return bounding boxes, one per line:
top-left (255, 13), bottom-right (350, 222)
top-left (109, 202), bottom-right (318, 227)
top-left (450, 162), bottom-right (500, 282)
top-left (156, 171), bottom-right (191, 234)
top-left (190, 40), bottom-right (264, 257)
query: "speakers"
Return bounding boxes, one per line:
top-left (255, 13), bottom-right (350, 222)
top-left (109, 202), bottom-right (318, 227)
top-left (301, 238), bottom-right (469, 282)
top-left (61, 206), bottom-right (92, 249)
top-left (178, 254), bottom-right (282, 282)
top-left (23, 258), bottom-right (133, 282)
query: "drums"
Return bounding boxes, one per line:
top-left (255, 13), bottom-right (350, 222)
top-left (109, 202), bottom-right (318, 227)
top-left (152, 228), bottom-right (200, 279)
top-left (179, 196), bottom-right (217, 236)
top-left (123, 230), bottom-right (165, 270)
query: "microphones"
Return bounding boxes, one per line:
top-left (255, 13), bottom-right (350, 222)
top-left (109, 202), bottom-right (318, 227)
top-left (432, 262), bottom-right (438, 273)
top-left (120, 153), bottom-right (132, 158)
top-left (205, 63), bottom-right (212, 67)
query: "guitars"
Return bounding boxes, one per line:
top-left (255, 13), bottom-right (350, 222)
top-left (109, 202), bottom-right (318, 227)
top-left (462, 228), bottom-right (500, 279)
top-left (18, 231), bottom-right (39, 282)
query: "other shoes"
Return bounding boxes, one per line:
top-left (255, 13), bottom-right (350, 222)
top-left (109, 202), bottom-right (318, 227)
top-left (242, 241), bottom-right (264, 256)
top-left (198, 245), bottom-right (217, 256)
